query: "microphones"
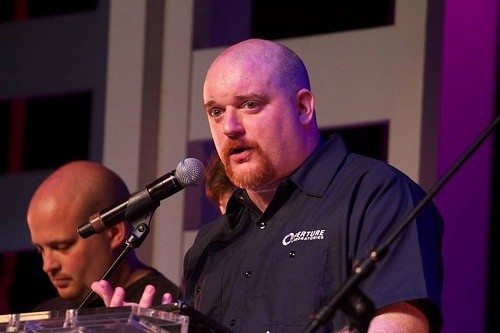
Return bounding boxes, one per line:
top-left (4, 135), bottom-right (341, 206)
top-left (77, 157), bottom-right (207, 240)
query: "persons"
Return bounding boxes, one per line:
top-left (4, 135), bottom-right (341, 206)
top-left (27, 161), bottom-right (180, 313)
top-left (205, 157), bottom-right (240, 214)
top-left (90, 38), bottom-right (446, 333)
